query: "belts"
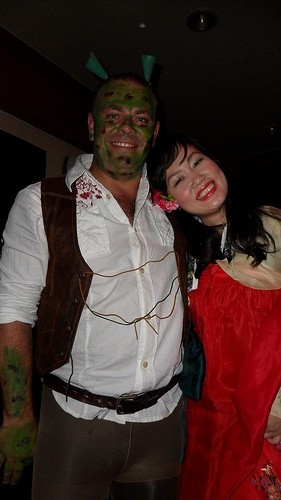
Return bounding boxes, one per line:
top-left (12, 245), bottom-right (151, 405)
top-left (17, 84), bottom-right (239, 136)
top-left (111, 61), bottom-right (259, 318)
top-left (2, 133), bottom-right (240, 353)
top-left (42, 373), bottom-right (178, 415)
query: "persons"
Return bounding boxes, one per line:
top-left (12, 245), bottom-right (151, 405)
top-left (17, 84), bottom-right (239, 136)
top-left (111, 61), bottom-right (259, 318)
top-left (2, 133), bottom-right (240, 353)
top-left (145, 131), bottom-right (281, 500)
top-left (0, 74), bottom-right (189, 500)
